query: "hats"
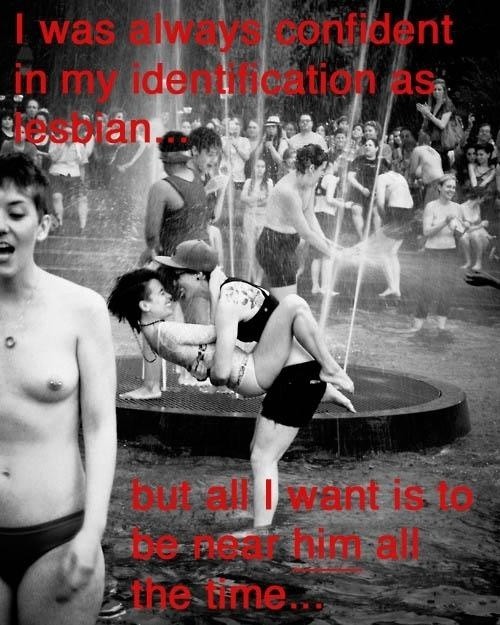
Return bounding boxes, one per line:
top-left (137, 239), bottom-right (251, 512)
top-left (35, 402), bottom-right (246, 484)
top-left (264, 116), bottom-right (280, 126)
top-left (155, 240), bottom-right (218, 270)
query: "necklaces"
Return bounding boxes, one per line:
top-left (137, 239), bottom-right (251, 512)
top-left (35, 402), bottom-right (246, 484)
top-left (2, 268), bottom-right (41, 349)
top-left (131, 318), bottom-right (166, 329)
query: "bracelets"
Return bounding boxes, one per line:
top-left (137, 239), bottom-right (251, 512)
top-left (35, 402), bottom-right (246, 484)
top-left (430, 115), bottom-right (436, 120)
top-left (232, 143), bottom-right (239, 150)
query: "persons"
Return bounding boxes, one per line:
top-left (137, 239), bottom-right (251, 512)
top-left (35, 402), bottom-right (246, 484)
top-left (108, 268), bottom-right (357, 413)
top-left (154, 240), bottom-right (329, 540)
top-left (380, 125), bottom-right (416, 175)
top-left (239, 160), bottom-right (273, 285)
top-left (444, 108), bottom-right (499, 199)
top-left (415, 78), bottom-right (455, 148)
top-left (53, 108), bottom-right (126, 145)
top-left (370, 159), bottom-right (415, 298)
top-left (0, 93), bottom-right (51, 170)
top-left (220, 117), bottom-right (250, 189)
top-left (285, 122), bottom-right (297, 138)
top-left (255, 144), bottom-right (343, 304)
top-left (347, 139), bottom-right (386, 240)
top-left (190, 127), bottom-right (228, 224)
top-left (315, 116), bottom-right (365, 150)
top-left (409, 131), bottom-right (444, 185)
top-left (363, 121), bottom-right (393, 161)
top-left (48, 125), bottom-right (89, 237)
top-left (253, 115), bottom-right (288, 184)
top-left (311, 150), bottom-right (351, 295)
top-left (0, 153), bottom-right (120, 625)
top-left (109, 122), bottom-right (148, 174)
top-left (289, 115), bottom-right (328, 152)
top-left (180, 116), bottom-right (229, 136)
top-left (414, 175), bottom-right (470, 330)
top-left (459, 187), bottom-right (488, 270)
top-left (245, 120), bottom-right (260, 150)
top-left (119, 133), bottom-right (212, 400)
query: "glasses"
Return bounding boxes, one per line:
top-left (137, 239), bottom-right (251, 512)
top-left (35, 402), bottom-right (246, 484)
top-left (172, 271), bottom-right (187, 280)
